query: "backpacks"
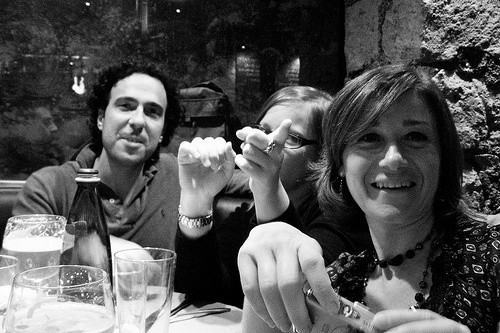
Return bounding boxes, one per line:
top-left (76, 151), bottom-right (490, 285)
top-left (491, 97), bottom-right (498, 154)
top-left (167, 80), bottom-right (241, 157)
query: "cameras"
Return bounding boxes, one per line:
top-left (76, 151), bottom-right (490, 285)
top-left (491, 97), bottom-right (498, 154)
top-left (304, 284), bottom-right (377, 333)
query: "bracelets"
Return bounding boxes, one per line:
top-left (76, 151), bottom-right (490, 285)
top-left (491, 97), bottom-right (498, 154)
top-left (177, 206), bottom-right (213, 228)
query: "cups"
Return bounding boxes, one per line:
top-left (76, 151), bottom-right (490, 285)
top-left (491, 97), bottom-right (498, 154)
top-left (0, 254), bottom-right (20, 333)
top-left (112, 247), bottom-right (177, 333)
top-left (3, 266), bottom-right (116, 333)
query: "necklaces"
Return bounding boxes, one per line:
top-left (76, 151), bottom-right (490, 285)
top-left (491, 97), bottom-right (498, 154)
top-left (369, 222), bottom-right (437, 306)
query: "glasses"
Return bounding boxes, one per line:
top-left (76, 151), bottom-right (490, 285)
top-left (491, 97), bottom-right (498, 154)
top-left (253, 124), bottom-right (317, 150)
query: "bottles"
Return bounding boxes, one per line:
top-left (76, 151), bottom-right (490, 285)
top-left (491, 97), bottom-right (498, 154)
top-left (58, 167), bottom-right (113, 307)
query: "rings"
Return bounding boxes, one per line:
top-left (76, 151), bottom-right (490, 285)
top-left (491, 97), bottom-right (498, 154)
top-left (263, 139), bottom-right (276, 154)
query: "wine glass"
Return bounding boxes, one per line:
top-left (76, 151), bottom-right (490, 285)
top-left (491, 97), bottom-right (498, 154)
top-left (2, 213), bottom-right (66, 301)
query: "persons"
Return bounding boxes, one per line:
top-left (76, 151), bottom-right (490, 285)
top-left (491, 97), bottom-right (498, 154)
top-left (174, 86), bottom-right (335, 311)
top-left (12, 63), bottom-right (182, 286)
top-left (237, 64), bottom-right (500, 333)
